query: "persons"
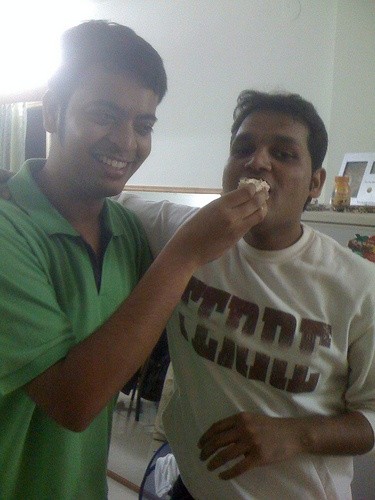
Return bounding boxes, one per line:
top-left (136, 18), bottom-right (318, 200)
top-left (0, 20), bottom-right (268, 496)
top-left (2, 89), bottom-right (373, 496)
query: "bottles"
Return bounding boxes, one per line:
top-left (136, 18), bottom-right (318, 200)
top-left (331, 176), bottom-right (351, 212)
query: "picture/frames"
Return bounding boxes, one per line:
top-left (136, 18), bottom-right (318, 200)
top-left (330, 152), bottom-right (374, 205)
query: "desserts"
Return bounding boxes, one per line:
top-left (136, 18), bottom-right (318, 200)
top-left (238, 177), bottom-right (271, 192)
